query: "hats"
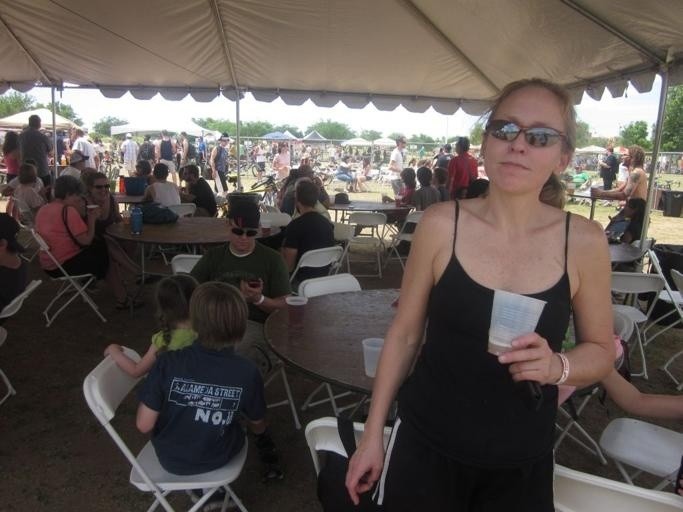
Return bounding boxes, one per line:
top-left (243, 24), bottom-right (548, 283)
top-left (69, 150), bottom-right (89, 165)
top-left (0, 212), bottom-right (27, 253)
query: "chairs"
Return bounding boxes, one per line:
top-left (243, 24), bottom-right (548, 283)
top-left (29, 226), bottom-right (111, 330)
top-left (169, 252), bottom-right (208, 281)
top-left (657, 269), bottom-right (683, 389)
top-left (260, 363), bottom-right (302, 430)
top-left (303, 418), bottom-right (393, 484)
top-left (287, 244), bottom-right (343, 294)
top-left (296, 271), bottom-right (384, 418)
top-left (549, 309), bottom-right (636, 468)
top-left (552, 459), bottom-right (682, 512)
top-left (343, 212), bottom-right (386, 282)
top-left (384, 209), bottom-right (423, 276)
top-left (598, 414), bottom-right (683, 491)
top-left (145, 201), bottom-right (195, 269)
top-left (643, 246), bottom-right (681, 351)
top-left (604, 268), bottom-right (656, 381)
top-left (9, 194), bottom-right (51, 263)
top-left (0, 278), bottom-right (44, 407)
top-left (83, 346), bottom-right (249, 511)
top-left (332, 216), bottom-right (358, 276)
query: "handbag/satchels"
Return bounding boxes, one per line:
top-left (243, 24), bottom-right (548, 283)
top-left (61, 204), bottom-right (106, 265)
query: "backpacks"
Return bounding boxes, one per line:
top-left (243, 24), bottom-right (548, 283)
top-left (187, 140), bottom-right (197, 159)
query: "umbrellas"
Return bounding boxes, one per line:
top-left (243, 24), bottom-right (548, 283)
top-left (609, 144), bottom-right (627, 158)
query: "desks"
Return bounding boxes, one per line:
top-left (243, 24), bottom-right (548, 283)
top-left (106, 214), bottom-right (282, 287)
top-left (263, 286), bottom-right (441, 417)
top-left (325, 199), bottom-right (408, 223)
top-left (566, 185), bottom-right (623, 225)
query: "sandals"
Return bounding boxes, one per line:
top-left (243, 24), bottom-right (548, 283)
top-left (134, 272), bottom-right (161, 283)
top-left (116, 296), bottom-right (145, 309)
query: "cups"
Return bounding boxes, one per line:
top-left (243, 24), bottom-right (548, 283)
top-left (395, 196), bottom-right (402, 206)
top-left (128, 208), bottom-right (144, 240)
top-left (122, 211), bottom-right (131, 224)
top-left (487, 288), bottom-right (547, 357)
top-left (87, 205), bottom-right (99, 213)
top-left (286, 296), bottom-right (307, 336)
top-left (362, 338), bottom-right (385, 378)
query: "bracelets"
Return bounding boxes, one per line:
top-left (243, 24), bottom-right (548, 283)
top-left (254, 295), bottom-right (266, 306)
top-left (554, 353), bottom-right (570, 385)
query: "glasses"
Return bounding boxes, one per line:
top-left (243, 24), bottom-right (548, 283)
top-left (488, 117), bottom-right (576, 150)
top-left (229, 226), bottom-right (259, 237)
top-left (92, 184), bottom-right (111, 189)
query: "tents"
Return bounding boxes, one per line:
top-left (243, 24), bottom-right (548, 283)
top-left (370, 136), bottom-right (396, 147)
top-left (341, 137), bottom-right (370, 147)
top-left (573, 144), bottom-right (606, 155)
top-left (299, 129), bottom-right (333, 144)
top-left (284, 130), bottom-right (299, 141)
top-left (262, 132), bottom-right (289, 140)
top-left (110, 108), bottom-right (235, 144)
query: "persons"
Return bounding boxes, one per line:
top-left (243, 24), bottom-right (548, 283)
top-left (179, 165), bottom-right (218, 217)
top-left (346, 76), bottom-right (616, 511)
top-left (390, 135), bottom-right (490, 208)
top-left (0, 114), bottom-right (123, 310)
top-left (136, 282), bottom-right (269, 512)
top-left (189, 200), bottom-right (294, 369)
top-left (274, 166), bottom-right (336, 283)
top-left (232, 138), bottom-right (334, 200)
top-left (599, 365), bottom-right (682, 495)
top-left (179, 131), bottom-right (195, 167)
top-left (591, 145), bottom-right (648, 243)
top-left (210, 132), bottom-right (231, 193)
top-left (198, 136), bottom-right (206, 164)
top-left (598, 141), bottom-right (618, 190)
top-left (570, 166), bottom-right (591, 193)
top-left (103, 273), bottom-right (199, 379)
top-left (157, 129), bottom-right (177, 184)
top-left (327, 146), bottom-right (390, 192)
top-left (120, 132), bottom-right (139, 177)
top-left (142, 163), bottom-right (183, 219)
top-left (140, 134), bottom-right (156, 164)
